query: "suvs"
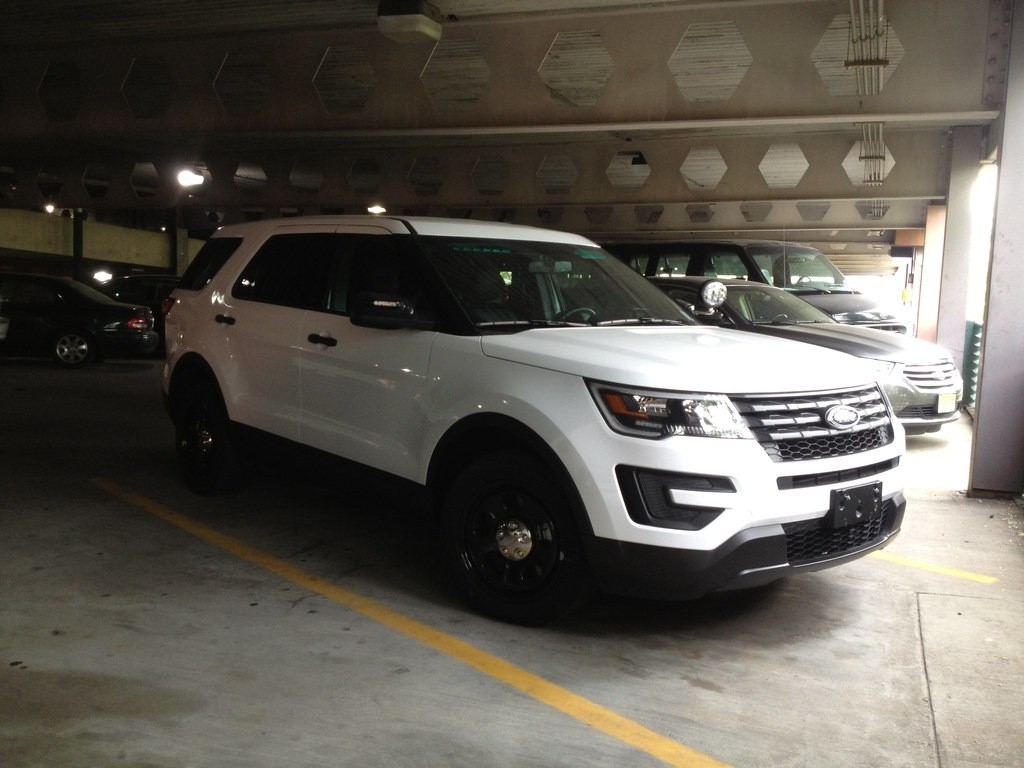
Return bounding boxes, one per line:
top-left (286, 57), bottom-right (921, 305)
top-left (160, 215), bottom-right (908, 630)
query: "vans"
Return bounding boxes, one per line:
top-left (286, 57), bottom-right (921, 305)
top-left (589, 238), bottom-right (906, 337)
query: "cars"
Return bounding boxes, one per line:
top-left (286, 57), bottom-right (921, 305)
top-left (1, 274), bottom-right (180, 373)
top-left (626, 274), bottom-right (963, 435)
